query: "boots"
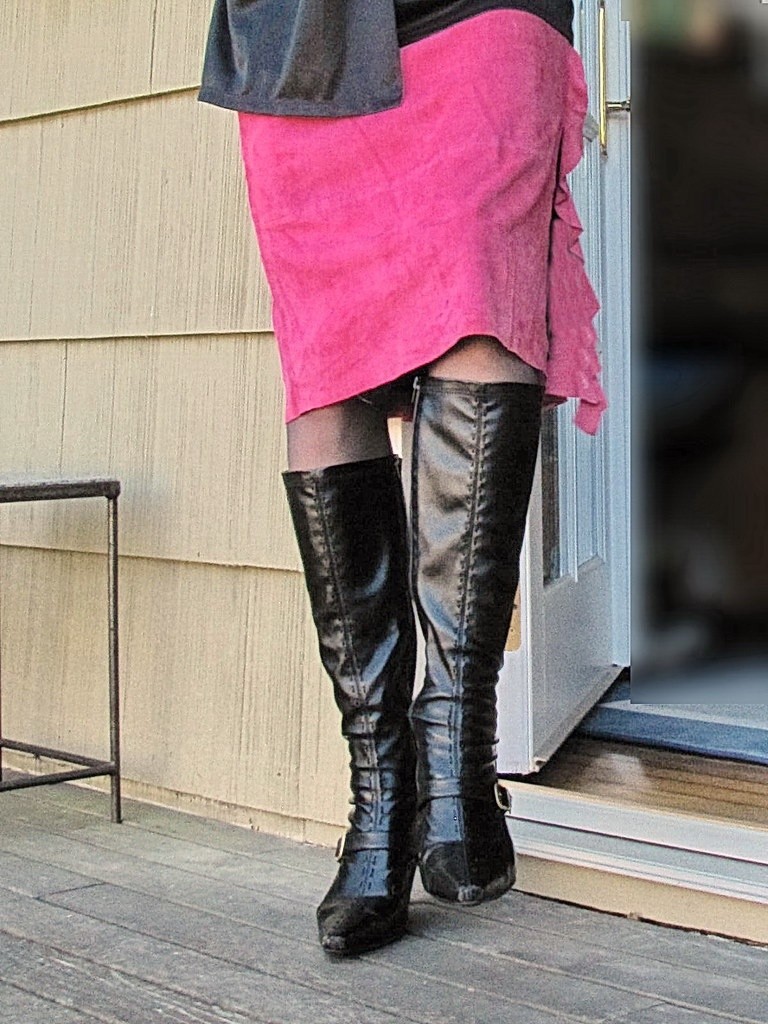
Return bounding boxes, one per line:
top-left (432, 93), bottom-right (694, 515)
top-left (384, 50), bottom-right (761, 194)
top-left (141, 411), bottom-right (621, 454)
top-left (406, 374), bottom-right (547, 906)
top-left (281, 454), bottom-right (419, 957)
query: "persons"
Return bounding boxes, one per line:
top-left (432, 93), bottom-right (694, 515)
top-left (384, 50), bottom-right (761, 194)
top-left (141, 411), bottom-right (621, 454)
top-left (198, 0), bottom-right (609, 958)
